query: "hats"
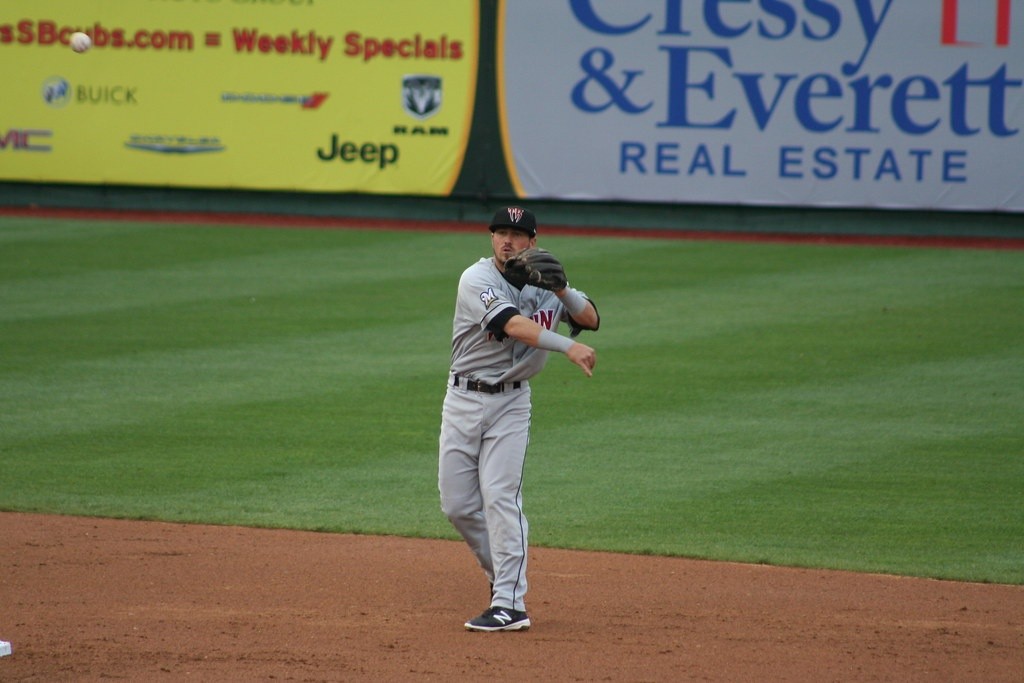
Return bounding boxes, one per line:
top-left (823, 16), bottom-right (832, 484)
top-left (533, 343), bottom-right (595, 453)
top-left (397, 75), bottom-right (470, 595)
top-left (489, 207), bottom-right (537, 238)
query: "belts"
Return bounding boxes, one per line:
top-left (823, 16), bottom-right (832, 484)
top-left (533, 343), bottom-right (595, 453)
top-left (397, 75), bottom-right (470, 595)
top-left (454, 376), bottom-right (520, 393)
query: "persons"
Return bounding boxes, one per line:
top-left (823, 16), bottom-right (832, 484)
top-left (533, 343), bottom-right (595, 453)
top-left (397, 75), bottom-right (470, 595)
top-left (437, 209), bottom-right (600, 631)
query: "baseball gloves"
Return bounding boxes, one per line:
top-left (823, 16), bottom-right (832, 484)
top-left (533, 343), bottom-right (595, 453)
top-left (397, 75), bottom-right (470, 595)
top-left (504, 247), bottom-right (567, 291)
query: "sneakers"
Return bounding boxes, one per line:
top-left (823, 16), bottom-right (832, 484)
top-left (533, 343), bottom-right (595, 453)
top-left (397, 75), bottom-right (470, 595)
top-left (464, 606), bottom-right (531, 632)
top-left (489, 582), bottom-right (493, 606)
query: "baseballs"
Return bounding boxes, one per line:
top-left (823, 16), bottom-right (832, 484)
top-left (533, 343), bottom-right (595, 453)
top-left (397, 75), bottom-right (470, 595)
top-left (69, 32), bottom-right (92, 53)
